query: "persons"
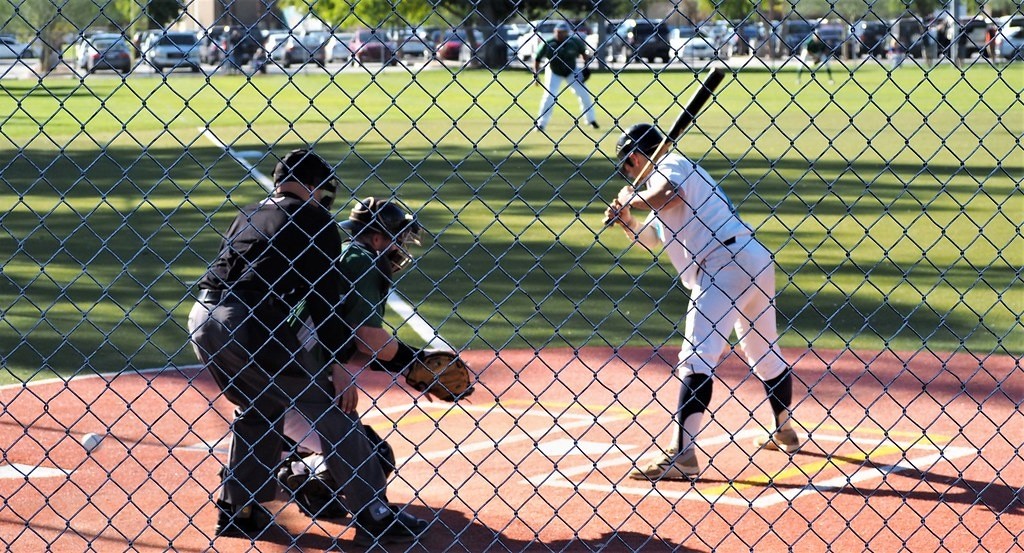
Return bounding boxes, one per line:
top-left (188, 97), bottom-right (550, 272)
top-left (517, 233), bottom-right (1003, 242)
top-left (187, 146), bottom-right (474, 547)
top-left (533, 21), bottom-right (600, 128)
top-left (604, 124), bottom-right (800, 481)
top-left (796, 29), bottom-right (835, 87)
top-left (923, 19), bottom-right (945, 70)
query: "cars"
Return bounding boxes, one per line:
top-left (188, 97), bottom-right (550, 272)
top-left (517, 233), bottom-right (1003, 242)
top-left (0, 37), bottom-right (34, 59)
top-left (388, 13), bottom-right (1024, 62)
top-left (129, 25), bottom-right (223, 74)
top-left (348, 29), bottom-right (396, 67)
top-left (324, 32), bottom-right (354, 63)
top-left (260, 27), bottom-right (331, 60)
top-left (74, 30), bottom-right (132, 74)
top-left (281, 36), bottom-right (326, 67)
top-left (213, 24), bottom-right (268, 73)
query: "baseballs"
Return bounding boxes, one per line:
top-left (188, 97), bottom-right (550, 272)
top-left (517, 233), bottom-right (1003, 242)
top-left (81, 433), bottom-right (101, 452)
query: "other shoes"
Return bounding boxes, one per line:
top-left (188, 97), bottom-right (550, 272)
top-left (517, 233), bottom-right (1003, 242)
top-left (587, 120), bottom-right (599, 129)
top-left (534, 124), bottom-right (545, 131)
top-left (795, 80), bottom-right (801, 86)
top-left (827, 79), bottom-right (835, 86)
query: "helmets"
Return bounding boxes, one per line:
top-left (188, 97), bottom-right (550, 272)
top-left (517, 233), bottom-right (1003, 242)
top-left (348, 197), bottom-right (419, 235)
top-left (274, 148), bottom-right (337, 192)
top-left (553, 23), bottom-right (570, 31)
top-left (615, 123), bottom-right (666, 180)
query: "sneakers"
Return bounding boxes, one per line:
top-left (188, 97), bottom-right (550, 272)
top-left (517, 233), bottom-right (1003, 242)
top-left (753, 428), bottom-right (800, 452)
top-left (352, 503), bottom-right (431, 546)
top-left (277, 461), bottom-right (330, 519)
top-left (629, 448), bottom-right (701, 479)
top-left (214, 500), bottom-right (288, 539)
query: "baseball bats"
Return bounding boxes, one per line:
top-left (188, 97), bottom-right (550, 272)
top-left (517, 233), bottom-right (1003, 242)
top-left (602, 67), bottom-right (725, 227)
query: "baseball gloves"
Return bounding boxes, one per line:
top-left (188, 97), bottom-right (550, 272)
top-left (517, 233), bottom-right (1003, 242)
top-left (406, 352), bottom-right (475, 403)
top-left (580, 68), bottom-right (591, 84)
